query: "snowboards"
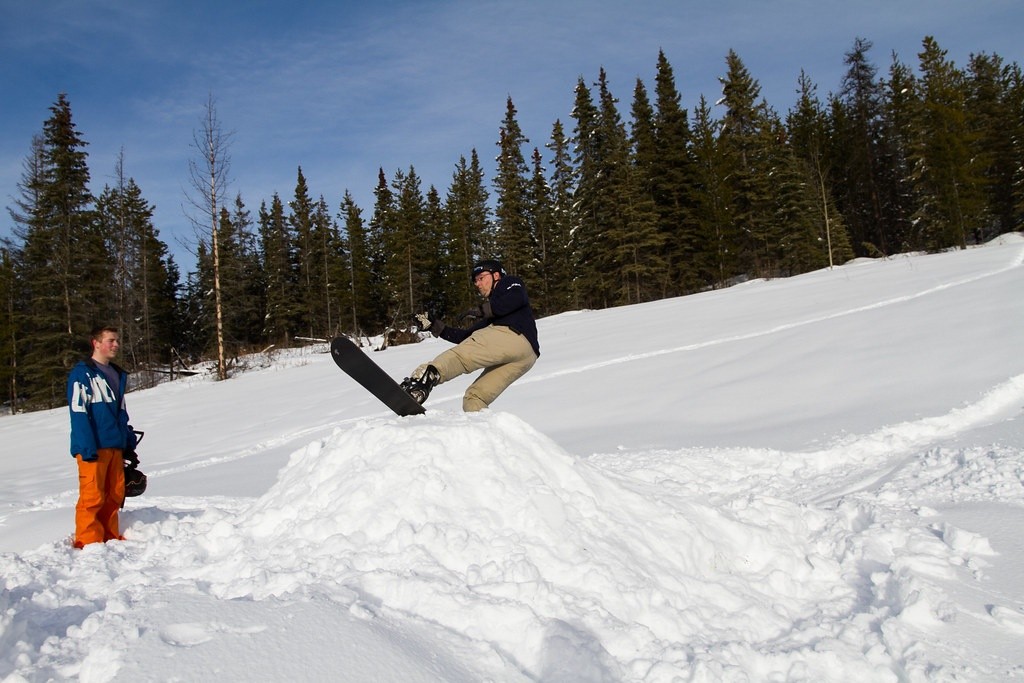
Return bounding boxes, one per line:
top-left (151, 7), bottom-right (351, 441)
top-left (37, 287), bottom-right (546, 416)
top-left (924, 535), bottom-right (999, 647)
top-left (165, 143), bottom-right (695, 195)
top-left (330, 333), bottom-right (424, 419)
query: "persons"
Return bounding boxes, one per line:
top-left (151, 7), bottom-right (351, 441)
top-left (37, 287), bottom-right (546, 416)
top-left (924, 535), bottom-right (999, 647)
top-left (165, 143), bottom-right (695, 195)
top-left (66, 324), bottom-right (131, 550)
top-left (399, 259), bottom-right (541, 414)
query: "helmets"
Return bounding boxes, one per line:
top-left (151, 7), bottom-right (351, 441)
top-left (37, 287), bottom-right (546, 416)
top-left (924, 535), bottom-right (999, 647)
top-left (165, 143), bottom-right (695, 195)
top-left (473, 259), bottom-right (507, 276)
top-left (120, 466), bottom-right (147, 508)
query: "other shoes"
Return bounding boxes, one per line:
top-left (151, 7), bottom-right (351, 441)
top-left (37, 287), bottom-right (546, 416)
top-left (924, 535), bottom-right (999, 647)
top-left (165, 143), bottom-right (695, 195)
top-left (399, 364), bottom-right (441, 405)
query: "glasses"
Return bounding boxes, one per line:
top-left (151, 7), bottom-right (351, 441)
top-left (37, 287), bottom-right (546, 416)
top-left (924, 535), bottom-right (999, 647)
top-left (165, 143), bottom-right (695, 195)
top-left (473, 274), bottom-right (491, 285)
